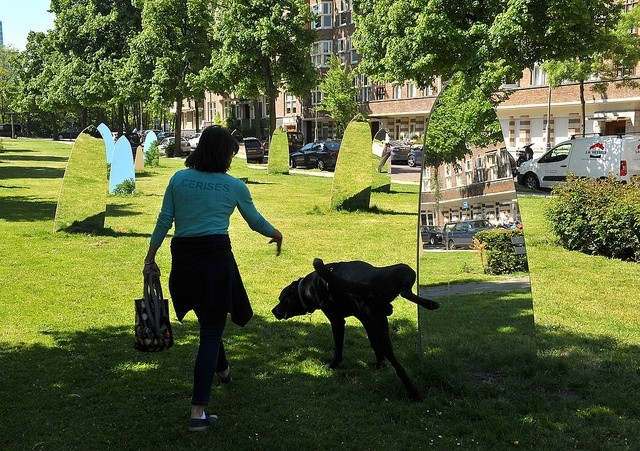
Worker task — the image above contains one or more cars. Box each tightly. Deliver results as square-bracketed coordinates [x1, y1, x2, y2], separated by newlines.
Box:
[158, 135, 191, 156]
[389, 138, 408, 163]
[119, 132, 140, 146]
[189, 132, 202, 148]
[290, 140, 340, 169]
[112, 131, 119, 141]
[58, 128, 79, 138]
[407, 143, 423, 166]
[420, 225, 443, 245]
[157, 131, 176, 144]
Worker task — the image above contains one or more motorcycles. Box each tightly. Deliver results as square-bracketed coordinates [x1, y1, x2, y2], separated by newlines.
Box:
[516, 141, 535, 164]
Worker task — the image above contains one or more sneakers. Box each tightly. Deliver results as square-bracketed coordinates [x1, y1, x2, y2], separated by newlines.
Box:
[216, 363, 233, 385]
[187, 410, 219, 432]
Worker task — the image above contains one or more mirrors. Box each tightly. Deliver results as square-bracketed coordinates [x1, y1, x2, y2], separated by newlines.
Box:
[225, 129, 248, 184]
[417, 72, 539, 402]
[331, 113, 372, 213]
[50, 125, 108, 236]
[97, 123, 114, 191]
[109, 135, 136, 197]
[268, 126, 289, 175]
[143, 131, 159, 167]
[371, 128, 392, 194]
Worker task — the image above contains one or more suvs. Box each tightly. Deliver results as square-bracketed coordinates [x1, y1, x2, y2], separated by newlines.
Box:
[244, 136, 265, 163]
[140, 129, 162, 141]
[285, 130, 306, 151]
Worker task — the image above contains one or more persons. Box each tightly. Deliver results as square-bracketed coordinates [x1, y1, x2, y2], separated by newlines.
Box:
[142, 123, 283, 432]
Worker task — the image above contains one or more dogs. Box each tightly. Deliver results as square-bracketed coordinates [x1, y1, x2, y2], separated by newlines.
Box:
[271, 257, 441, 404]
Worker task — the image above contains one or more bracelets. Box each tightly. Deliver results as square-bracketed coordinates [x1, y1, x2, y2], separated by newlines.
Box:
[144, 259, 155, 265]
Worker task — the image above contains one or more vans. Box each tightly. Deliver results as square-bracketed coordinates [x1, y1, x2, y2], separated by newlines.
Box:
[1, 124, 22, 138]
[443, 219, 495, 249]
[518, 131, 639, 191]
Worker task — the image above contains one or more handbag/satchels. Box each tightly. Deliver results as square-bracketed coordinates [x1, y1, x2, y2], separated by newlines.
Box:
[133, 271, 174, 353]
